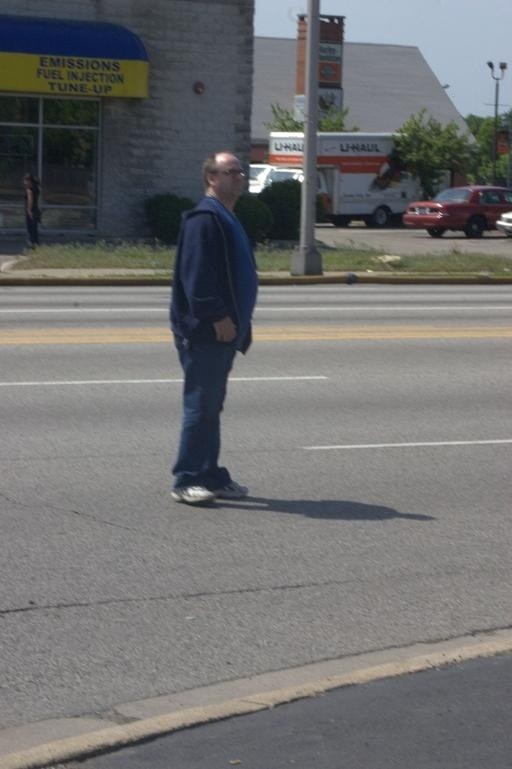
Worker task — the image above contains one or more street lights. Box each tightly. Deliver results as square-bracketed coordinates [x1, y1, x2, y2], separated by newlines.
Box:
[487, 59, 507, 185]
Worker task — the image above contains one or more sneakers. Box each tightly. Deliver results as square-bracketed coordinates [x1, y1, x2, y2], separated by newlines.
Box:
[213, 480, 249, 498]
[171, 486, 214, 504]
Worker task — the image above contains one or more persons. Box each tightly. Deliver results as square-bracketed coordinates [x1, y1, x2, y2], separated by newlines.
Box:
[21, 173, 42, 249]
[165, 150, 261, 510]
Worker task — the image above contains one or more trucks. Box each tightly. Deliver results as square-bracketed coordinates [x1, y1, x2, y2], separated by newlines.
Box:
[265, 128, 454, 229]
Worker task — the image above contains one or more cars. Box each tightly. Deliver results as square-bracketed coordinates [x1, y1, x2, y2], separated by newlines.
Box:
[403, 185, 510, 237]
[496, 210, 512, 236]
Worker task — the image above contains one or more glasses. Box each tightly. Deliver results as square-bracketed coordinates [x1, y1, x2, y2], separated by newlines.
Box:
[222, 169, 247, 177]
